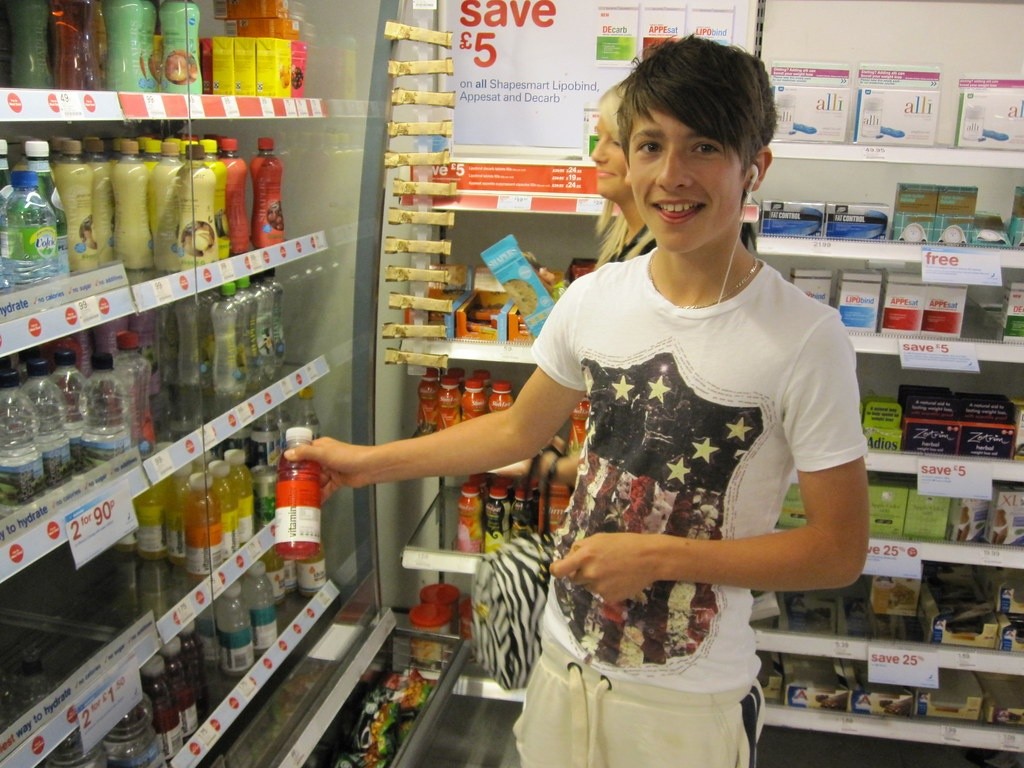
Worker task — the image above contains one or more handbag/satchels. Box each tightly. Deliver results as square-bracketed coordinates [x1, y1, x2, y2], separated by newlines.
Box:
[473, 532, 557, 691]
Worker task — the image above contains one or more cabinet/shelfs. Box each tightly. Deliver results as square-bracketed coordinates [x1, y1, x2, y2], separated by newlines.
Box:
[399, 128, 1023, 756]
[0, 85, 401, 768]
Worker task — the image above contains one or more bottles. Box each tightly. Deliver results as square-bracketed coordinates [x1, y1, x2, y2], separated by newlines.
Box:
[4, 0, 208, 95]
[0, 265, 305, 523]
[0, 136, 286, 286]
[0, 399, 331, 767]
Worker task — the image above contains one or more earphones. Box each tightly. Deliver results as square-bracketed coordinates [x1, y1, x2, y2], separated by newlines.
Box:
[747, 164, 759, 204]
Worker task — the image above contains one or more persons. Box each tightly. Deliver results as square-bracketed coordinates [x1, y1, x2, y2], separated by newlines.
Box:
[446, 74, 665, 497]
[276, 34, 869, 768]
